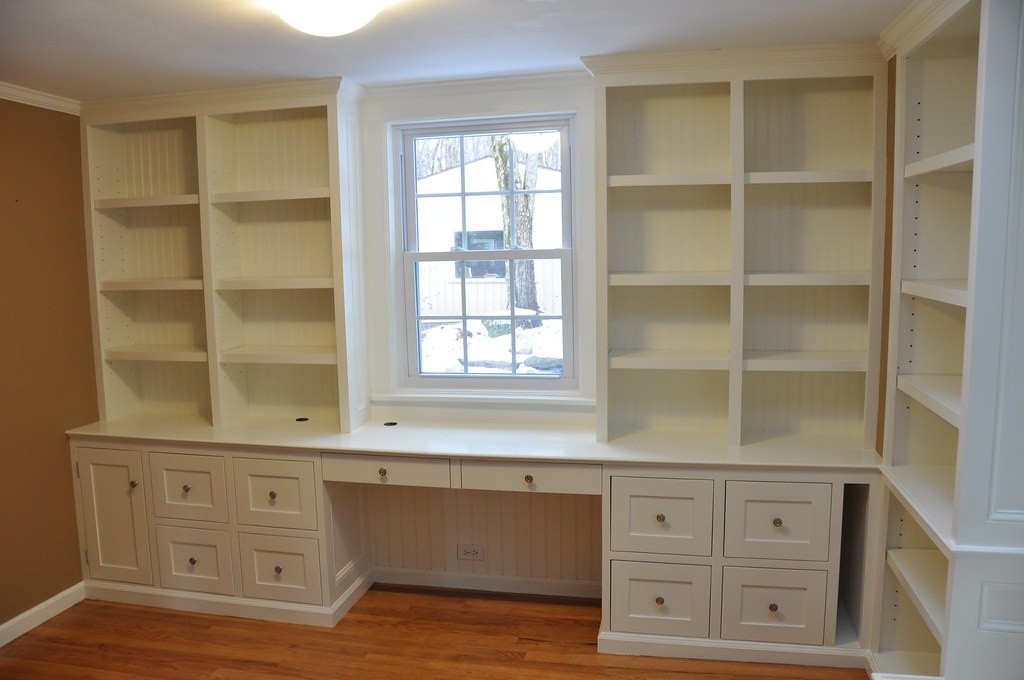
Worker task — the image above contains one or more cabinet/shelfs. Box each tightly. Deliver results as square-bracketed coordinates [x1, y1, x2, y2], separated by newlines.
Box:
[68, 436, 374, 629]
[593, 62, 888, 452]
[319, 423, 601, 496]
[602, 475, 845, 647]
[868, 0, 1023, 680]
[79, 92, 372, 434]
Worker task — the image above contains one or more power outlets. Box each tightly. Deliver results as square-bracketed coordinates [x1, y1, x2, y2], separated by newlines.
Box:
[458, 544, 485, 561]
